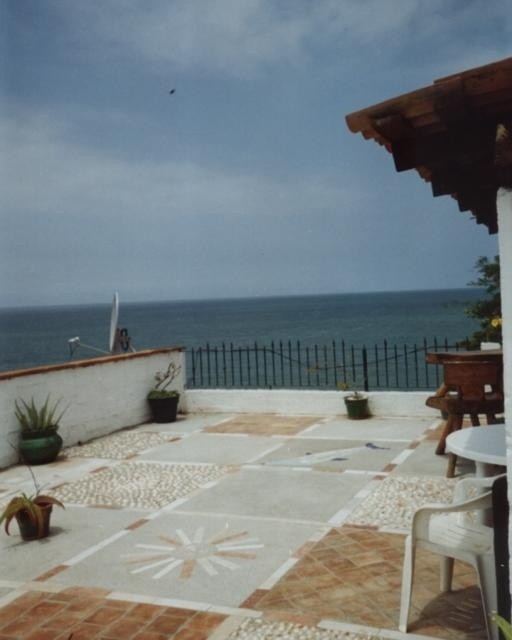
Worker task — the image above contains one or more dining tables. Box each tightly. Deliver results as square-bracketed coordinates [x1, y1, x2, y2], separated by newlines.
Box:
[0, 482, 66, 542]
[147, 360, 182, 423]
[4, 393, 72, 464]
[337, 375, 370, 420]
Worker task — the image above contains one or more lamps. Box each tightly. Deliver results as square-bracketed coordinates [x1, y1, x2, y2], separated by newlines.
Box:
[399, 473, 507, 640]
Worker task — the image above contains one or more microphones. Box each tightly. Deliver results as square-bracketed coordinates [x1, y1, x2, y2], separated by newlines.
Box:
[425, 394, 504, 478]
